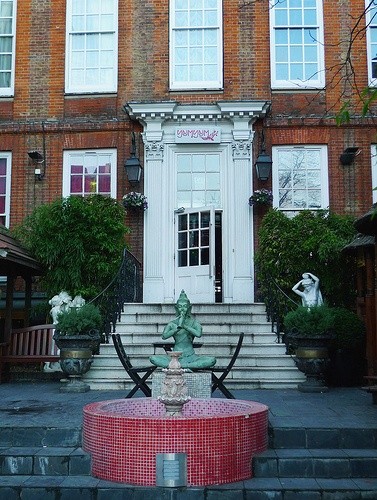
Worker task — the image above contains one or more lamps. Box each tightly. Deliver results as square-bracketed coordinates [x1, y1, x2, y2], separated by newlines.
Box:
[28, 150, 42, 162]
[124, 122, 144, 185]
[255, 119, 273, 183]
[340, 145, 361, 165]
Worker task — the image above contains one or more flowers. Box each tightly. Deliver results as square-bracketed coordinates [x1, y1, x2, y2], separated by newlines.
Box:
[248, 189, 274, 207]
[120, 193, 148, 210]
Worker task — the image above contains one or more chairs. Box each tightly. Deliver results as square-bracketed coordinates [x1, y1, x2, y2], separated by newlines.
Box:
[209, 332, 245, 399]
[111, 334, 157, 399]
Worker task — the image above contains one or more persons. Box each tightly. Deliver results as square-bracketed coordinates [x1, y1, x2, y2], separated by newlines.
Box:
[149, 289, 216, 369]
[43, 291, 86, 372]
[291, 272, 323, 311]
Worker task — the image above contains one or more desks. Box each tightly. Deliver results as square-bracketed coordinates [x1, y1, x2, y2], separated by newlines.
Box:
[153, 341, 205, 355]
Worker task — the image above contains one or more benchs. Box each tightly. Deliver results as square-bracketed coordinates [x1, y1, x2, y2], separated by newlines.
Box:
[1, 324, 60, 383]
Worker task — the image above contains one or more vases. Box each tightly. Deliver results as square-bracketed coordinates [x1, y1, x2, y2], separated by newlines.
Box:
[130, 203, 142, 212]
[256, 200, 269, 208]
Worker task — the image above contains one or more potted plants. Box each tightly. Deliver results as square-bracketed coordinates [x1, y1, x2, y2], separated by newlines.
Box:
[283, 305, 365, 392]
[55, 303, 104, 393]
